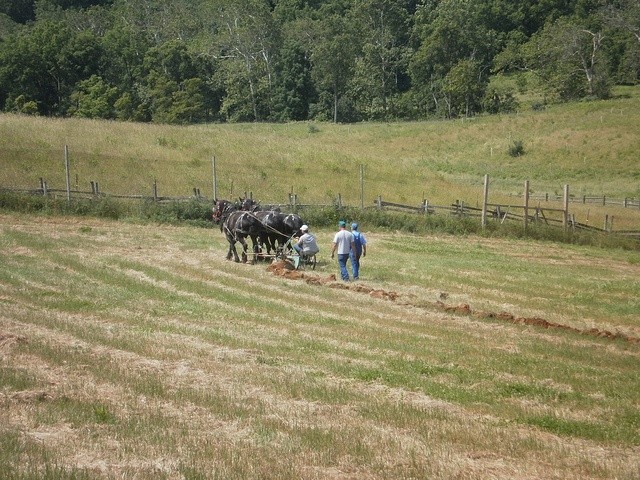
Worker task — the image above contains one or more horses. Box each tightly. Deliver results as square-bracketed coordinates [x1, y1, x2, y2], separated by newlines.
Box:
[212, 197, 261, 264]
[238, 195, 303, 254]
[235, 207, 284, 262]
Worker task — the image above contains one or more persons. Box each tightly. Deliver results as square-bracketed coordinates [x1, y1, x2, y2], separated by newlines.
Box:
[292, 224, 320, 260]
[330, 221, 357, 282]
[348, 222, 366, 279]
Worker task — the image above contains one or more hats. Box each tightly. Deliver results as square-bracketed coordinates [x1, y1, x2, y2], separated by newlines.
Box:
[351, 223, 359, 228]
[300, 225, 308, 230]
[338, 221, 345, 227]
